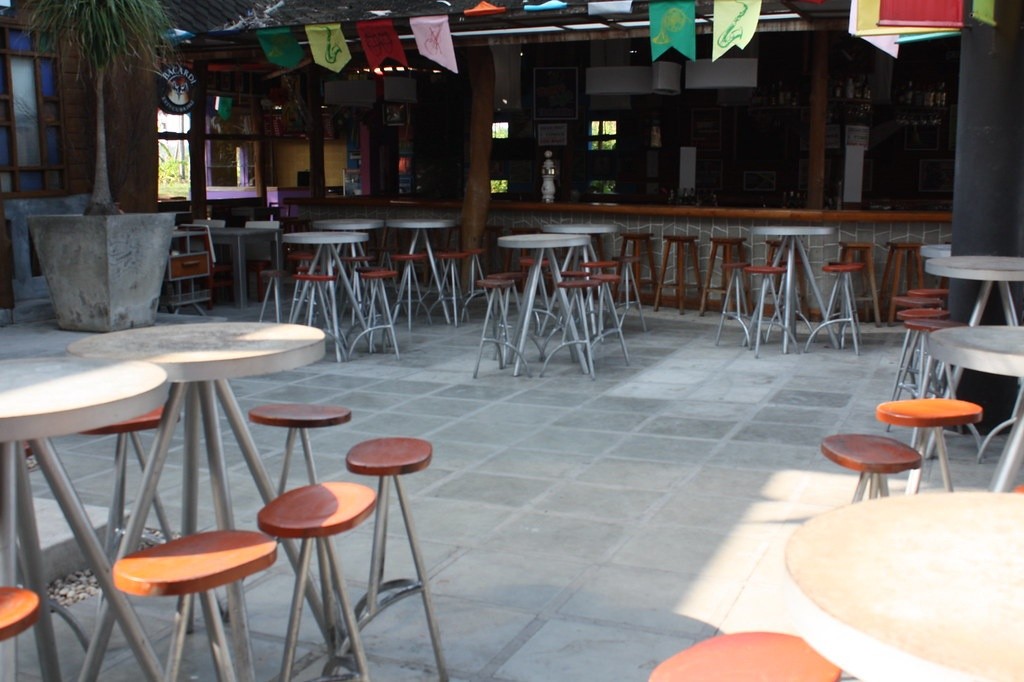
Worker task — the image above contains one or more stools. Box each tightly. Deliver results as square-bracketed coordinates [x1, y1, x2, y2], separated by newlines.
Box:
[0, 206, 985, 682]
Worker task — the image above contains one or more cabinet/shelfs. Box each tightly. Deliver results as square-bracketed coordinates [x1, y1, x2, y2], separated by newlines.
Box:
[161, 222, 216, 318]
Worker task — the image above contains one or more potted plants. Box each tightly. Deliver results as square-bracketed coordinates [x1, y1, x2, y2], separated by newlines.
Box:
[13, 1, 181, 331]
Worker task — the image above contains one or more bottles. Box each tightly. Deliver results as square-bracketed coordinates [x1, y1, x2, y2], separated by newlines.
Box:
[782, 191, 807, 208]
[825, 196, 836, 210]
[667, 188, 718, 206]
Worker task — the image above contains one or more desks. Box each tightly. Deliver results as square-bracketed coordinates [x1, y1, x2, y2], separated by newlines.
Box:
[919, 245, 952, 259]
[310, 218, 386, 327]
[497, 233, 591, 377]
[203, 186, 311, 222]
[539, 223, 618, 339]
[0, 358, 175, 682]
[742, 226, 840, 355]
[927, 325, 1024, 493]
[387, 220, 463, 326]
[67, 321, 337, 682]
[204, 228, 284, 310]
[778, 486, 1024, 682]
[281, 233, 377, 364]
[924, 256, 1023, 461]
